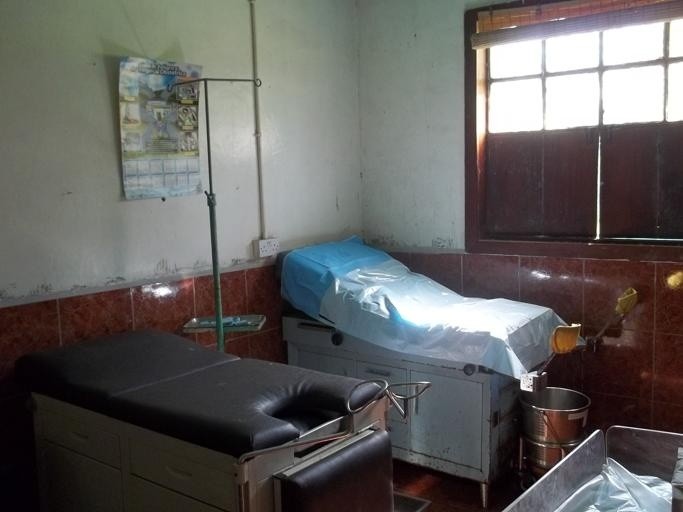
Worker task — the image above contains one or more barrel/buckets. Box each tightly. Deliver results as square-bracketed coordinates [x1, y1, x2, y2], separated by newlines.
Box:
[519, 386, 591, 474]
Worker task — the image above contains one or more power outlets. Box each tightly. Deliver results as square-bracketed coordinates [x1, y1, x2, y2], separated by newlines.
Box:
[253, 237, 288, 262]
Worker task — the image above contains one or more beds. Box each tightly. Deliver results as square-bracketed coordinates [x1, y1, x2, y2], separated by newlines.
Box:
[13, 319, 433, 512]
[274, 231, 640, 510]
